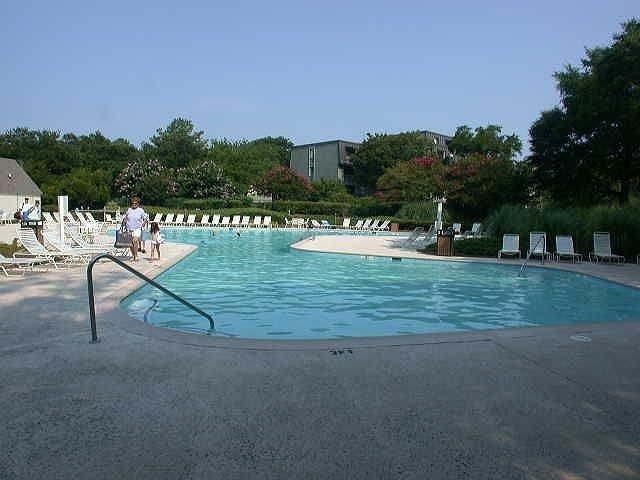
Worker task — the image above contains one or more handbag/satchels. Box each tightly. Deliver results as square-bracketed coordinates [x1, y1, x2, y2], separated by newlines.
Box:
[114, 229, 135, 249]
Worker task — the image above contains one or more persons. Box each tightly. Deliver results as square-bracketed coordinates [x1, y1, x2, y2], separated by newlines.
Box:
[21, 197, 32, 218]
[122, 197, 149, 263]
[149, 221, 164, 262]
[137, 214, 149, 254]
[27, 200, 40, 221]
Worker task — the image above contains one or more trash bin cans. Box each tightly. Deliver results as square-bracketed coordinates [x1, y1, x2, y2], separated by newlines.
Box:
[21, 219, 44, 246]
[437, 229, 455, 256]
[391, 223, 399, 232]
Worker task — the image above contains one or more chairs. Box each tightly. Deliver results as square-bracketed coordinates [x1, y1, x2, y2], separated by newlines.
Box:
[148, 213, 273, 229]
[0, 209, 131, 277]
[383, 225, 442, 251]
[283, 216, 336, 229]
[342, 218, 351, 228]
[497, 231, 626, 267]
[446, 222, 480, 240]
[352, 218, 391, 232]
